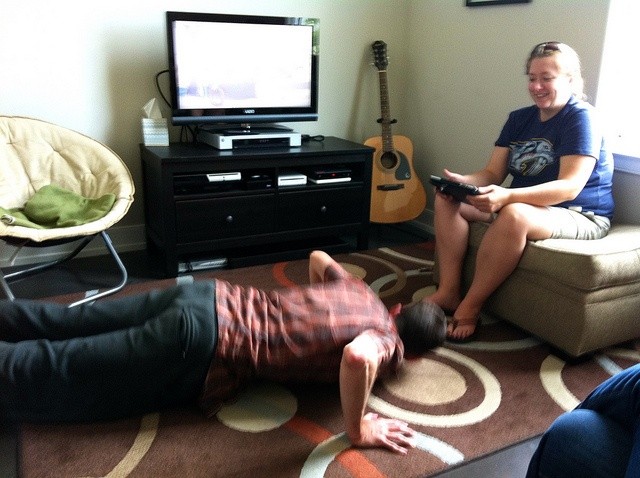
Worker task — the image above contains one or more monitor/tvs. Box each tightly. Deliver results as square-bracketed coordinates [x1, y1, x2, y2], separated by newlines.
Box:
[165, 11, 320, 132]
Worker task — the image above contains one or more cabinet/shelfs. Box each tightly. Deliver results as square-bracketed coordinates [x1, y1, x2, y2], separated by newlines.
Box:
[139, 136, 376, 276]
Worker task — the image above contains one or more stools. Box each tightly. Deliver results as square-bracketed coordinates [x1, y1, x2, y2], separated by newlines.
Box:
[433, 222, 640, 358]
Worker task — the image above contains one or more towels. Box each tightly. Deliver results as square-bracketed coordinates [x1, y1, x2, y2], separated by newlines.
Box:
[1, 185, 116, 229]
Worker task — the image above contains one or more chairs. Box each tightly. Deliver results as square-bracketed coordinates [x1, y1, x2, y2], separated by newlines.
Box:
[1, 117, 135, 308]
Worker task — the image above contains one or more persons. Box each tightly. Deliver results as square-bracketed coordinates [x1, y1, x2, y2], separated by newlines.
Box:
[1, 250, 448, 454]
[419, 41, 615, 344]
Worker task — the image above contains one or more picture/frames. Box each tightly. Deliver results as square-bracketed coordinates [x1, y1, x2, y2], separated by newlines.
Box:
[466, 0, 531, 6]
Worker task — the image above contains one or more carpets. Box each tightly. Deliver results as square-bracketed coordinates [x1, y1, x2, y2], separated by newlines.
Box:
[18, 240, 639, 477]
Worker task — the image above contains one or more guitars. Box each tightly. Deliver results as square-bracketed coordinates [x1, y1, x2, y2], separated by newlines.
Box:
[363, 39, 427, 225]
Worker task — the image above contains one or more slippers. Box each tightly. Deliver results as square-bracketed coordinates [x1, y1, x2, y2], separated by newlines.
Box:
[446, 315, 482, 344]
